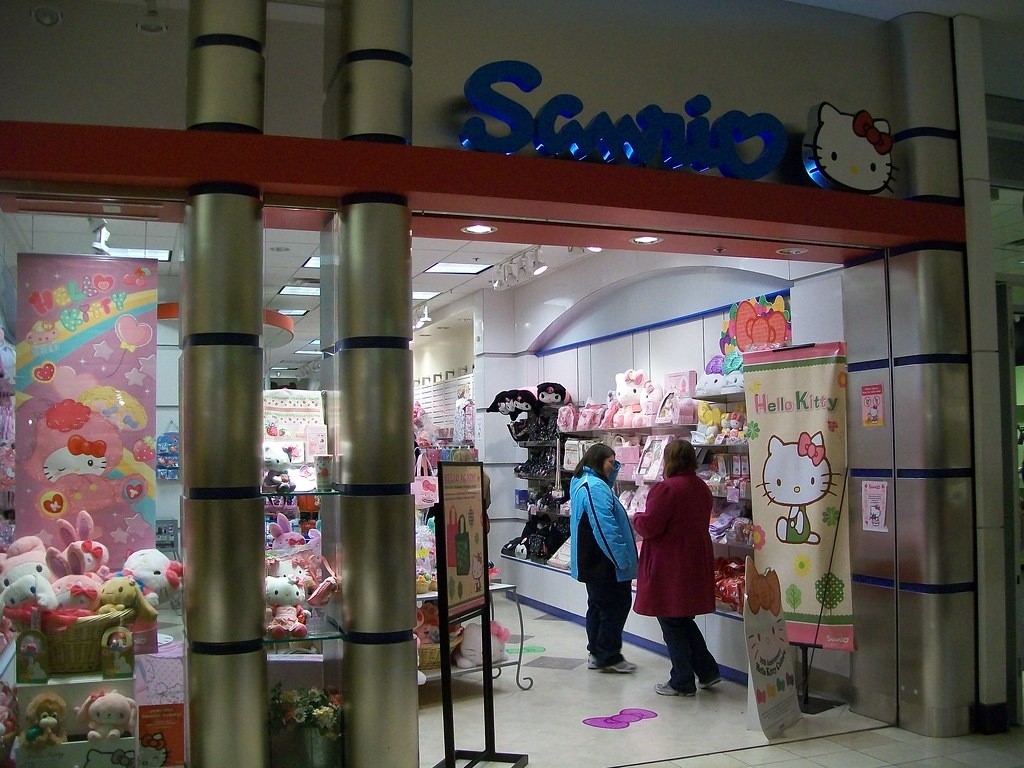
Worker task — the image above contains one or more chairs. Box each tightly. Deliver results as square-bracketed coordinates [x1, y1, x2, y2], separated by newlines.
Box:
[155, 520, 181, 562]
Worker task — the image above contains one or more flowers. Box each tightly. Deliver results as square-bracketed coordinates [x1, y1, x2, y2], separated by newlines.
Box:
[266, 682, 345, 742]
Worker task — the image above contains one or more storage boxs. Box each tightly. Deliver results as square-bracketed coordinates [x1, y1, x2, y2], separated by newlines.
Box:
[547, 536, 571, 569]
[613, 432, 681, 481]
[303, 425, 328, 463]
[564, 437, 602, 471]
[514, 489, 528, 509]
[643, 370, 696, 427]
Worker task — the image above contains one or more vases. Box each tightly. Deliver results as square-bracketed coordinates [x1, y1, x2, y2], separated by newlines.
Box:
[268, 726, 344, 768]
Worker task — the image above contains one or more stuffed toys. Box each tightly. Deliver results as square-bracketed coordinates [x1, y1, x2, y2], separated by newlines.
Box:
[19, 689, 138, 751]
[575, 369, 662, 430]
[705, 411, 748, 443]
[486, 382, 573, 564]
[263, 512, 324, 638]
[262, 446, 299, 493]
[695, 351, 745, 395]
[0, 509, 183, 621]
[412, 601, 510, 686]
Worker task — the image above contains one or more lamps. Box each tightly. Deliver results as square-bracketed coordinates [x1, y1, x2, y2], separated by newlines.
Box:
[492, 264, 502, 289]
[419, 304, 432, 322]
[532, 248, 547, 276]
[505, 259, 518, 287]
[90, 216, 112, 256]
[517, 252, 528, 282]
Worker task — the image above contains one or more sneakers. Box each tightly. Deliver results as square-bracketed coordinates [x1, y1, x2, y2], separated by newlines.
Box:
[587, 653, 599, 669]
[599, 660, 637, 673]
[697, 677, 721, 688]
[654, 682, 697, 697]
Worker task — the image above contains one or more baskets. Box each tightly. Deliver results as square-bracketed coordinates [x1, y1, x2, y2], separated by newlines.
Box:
[4, 602, 136, 673]
[419, 634, 465, 670]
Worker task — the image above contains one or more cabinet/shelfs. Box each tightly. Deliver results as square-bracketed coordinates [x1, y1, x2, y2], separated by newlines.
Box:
[518, 440, 570, 516]
[14, 671, 142, 767]
[263, 487, 345, 643]
[692, 388, 753, 547]
[553, 424, 697, 521]
[500, 549, 749, 686]
[416, 581, 534, 691]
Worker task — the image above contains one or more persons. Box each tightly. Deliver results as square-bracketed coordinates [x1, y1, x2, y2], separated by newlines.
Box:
[569, 442, 638, 673]
[630, 439, 722, 696]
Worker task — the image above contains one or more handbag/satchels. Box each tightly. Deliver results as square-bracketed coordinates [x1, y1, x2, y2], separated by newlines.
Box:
[447, 505, 459, 568]
[456, 514, 470, 575]
[412, 453, 439, 509]
